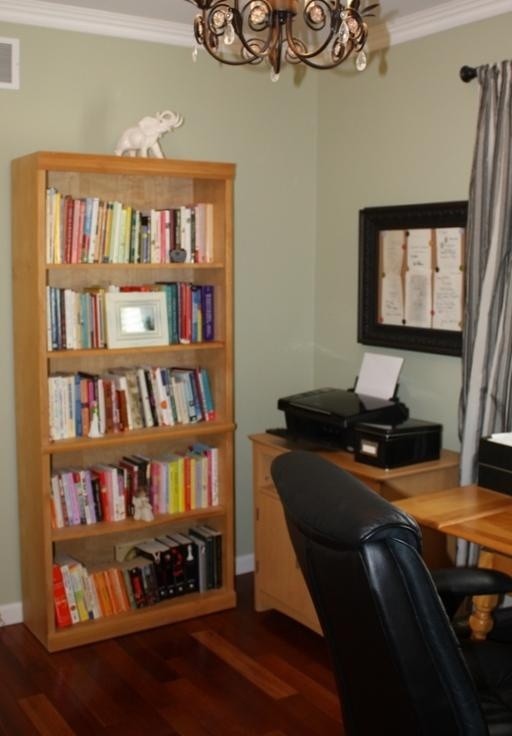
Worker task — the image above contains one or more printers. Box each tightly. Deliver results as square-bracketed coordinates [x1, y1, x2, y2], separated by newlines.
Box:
[276, 376, 408, 451]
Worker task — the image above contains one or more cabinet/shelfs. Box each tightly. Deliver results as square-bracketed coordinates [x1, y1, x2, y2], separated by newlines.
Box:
[248, 430, 469, 640]
[11, 149, 236, 655]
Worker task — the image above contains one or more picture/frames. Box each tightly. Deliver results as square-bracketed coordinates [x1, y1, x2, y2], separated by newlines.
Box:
[104, 291, 170, 351]
[356, 200, 468, 357]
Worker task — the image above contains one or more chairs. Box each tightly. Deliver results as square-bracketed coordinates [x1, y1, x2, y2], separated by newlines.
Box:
[270, 448, 511, 736]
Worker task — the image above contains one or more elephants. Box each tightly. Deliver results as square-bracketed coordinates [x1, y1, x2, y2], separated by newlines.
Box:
[114, 110, 184, 159]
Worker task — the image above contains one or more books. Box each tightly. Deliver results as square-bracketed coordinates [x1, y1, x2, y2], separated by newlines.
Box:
[51, 526, 223, 632]
[47, 366, 215, 440]
[45, 187, 215, 264]
[45, 281, 214, 352]
[50, 443, 220, 530]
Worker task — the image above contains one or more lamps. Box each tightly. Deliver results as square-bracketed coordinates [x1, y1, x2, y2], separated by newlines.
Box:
[185, 0, 379, 76]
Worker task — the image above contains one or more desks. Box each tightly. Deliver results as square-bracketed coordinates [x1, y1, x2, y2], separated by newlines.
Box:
[393, 484, 512, 643]
